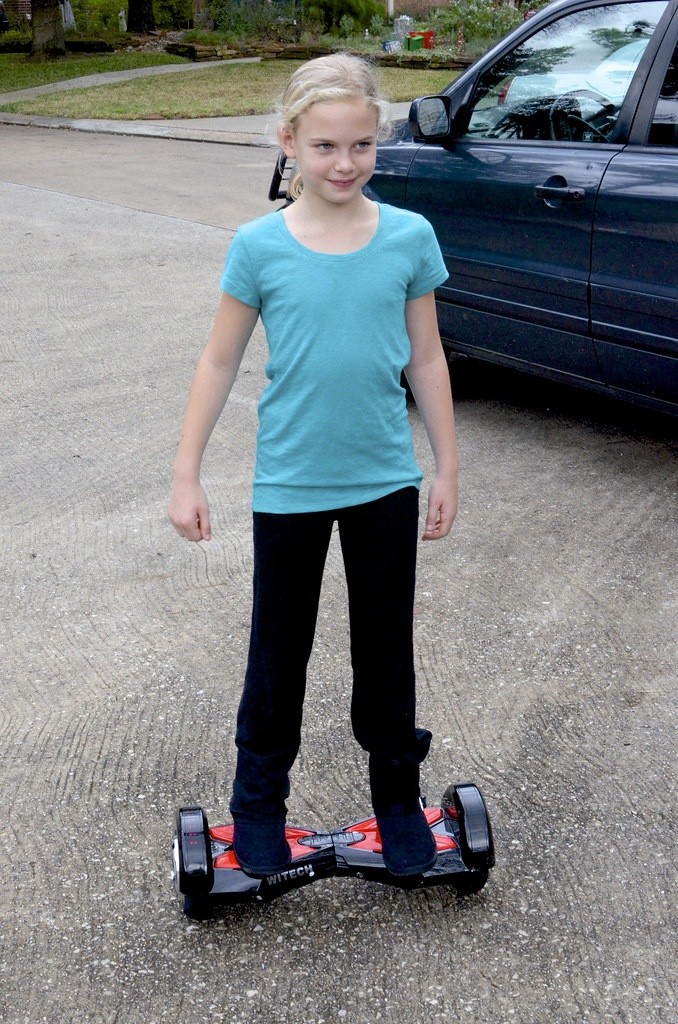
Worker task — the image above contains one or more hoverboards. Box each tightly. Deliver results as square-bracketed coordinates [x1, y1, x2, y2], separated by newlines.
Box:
[171, 779, 496, 928]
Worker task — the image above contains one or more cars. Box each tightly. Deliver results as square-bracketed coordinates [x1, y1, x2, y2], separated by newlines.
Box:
[268, 0, 678, 445]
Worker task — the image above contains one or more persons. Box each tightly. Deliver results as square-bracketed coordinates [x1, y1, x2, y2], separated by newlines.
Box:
[160, 55, 460, 883]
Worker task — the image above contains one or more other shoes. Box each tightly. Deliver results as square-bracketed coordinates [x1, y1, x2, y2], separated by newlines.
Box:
[375, 811, 438, 878]
[232, 822, 292, 878]
